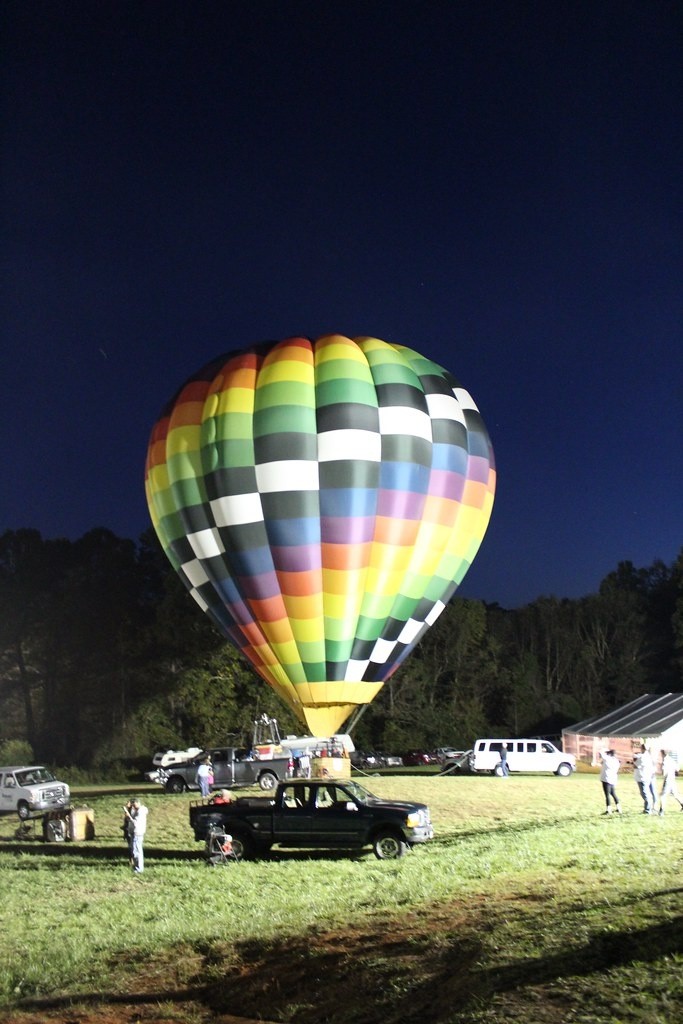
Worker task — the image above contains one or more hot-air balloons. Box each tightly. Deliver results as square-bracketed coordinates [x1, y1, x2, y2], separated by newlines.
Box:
[142, 331, 499, 781]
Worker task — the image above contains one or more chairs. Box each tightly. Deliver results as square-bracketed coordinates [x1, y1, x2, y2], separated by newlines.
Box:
[283, 791, 335, 808]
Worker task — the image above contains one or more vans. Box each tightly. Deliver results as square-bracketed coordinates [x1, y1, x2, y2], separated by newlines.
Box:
[0, 765, 71, 820]
[471, 738, 578, 777]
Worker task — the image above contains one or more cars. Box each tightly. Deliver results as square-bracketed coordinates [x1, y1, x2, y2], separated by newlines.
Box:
[441, 751, 474, 775]
[347, 745, 467, 770]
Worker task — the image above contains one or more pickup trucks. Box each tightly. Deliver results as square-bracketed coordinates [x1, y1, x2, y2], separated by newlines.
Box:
[144, 747, 295, 793]
[188, 779, 435, 861]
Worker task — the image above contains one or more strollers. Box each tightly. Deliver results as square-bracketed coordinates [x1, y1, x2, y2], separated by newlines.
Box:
[206, 822, 240, 869]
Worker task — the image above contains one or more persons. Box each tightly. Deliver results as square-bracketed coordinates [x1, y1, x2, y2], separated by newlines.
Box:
[500, 743, 509, 777]
[297, 747, 342, 778]
[195, 754, 215, 797]
[632, 744, 683, 817]
[598, 750, 624, 815]
[120, 798, 148, 872]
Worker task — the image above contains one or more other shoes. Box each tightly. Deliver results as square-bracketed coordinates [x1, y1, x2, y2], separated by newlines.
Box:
[643, 807, 665, 816]
[600, 808, 621, 815]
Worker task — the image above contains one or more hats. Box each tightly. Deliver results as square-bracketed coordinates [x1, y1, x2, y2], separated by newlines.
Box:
[130, 798, 140, 804]
[605, 749, 616, 755]
[641, 743, 649, 750]
